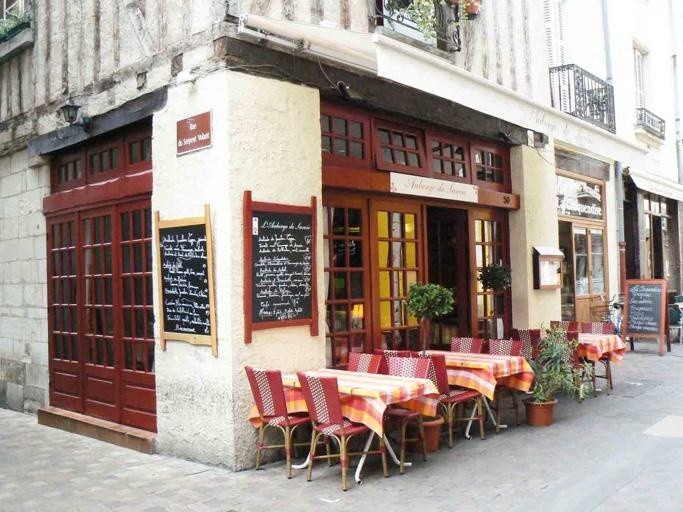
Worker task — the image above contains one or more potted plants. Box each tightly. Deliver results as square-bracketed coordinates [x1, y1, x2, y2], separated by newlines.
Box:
[666, 306, 682, 344]
[524, 331, 593, 428]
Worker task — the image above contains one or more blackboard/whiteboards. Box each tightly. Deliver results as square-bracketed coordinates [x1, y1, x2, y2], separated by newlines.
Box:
[154, 218, 216, 346]
[623, 279, 666, 338]
[242, 201, 318, 330]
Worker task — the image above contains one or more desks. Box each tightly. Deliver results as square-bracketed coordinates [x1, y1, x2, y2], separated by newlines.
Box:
[420, 349, 535, 439]
[275, 365, 439, 487]
[449, 321, 613, 434]
[541, 332, 628, 397]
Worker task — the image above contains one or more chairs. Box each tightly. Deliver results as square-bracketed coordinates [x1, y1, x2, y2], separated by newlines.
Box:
[348, 351, 383, 374]
[296, 372, 390, 492]
[380, 357, 431, 476]
[373, 348, 410, 375]
[243, 366, 332, 479]
[410, 352, 486, 449]
